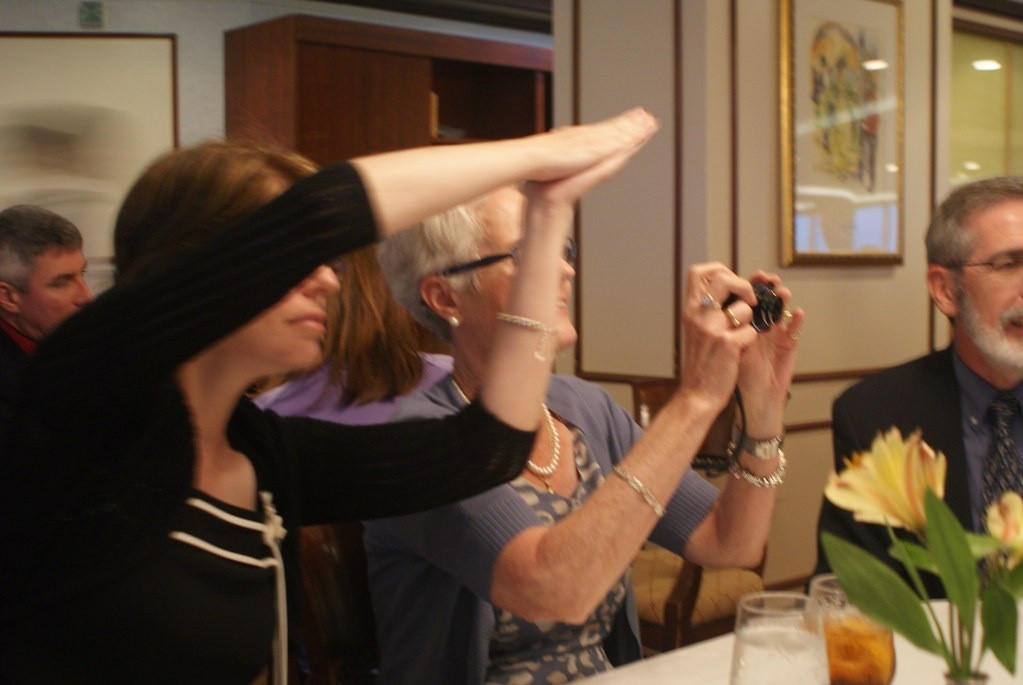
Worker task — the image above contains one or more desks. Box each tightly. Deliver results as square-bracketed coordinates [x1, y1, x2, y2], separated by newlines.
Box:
[560, 593, 1023, 685]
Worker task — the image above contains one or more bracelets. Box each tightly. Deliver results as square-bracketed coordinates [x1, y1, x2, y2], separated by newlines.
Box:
[724, 442, 785, 488]
[612, 465, 666, 517]
[735, 425, 784, 459]
[494, 313, 557, 334]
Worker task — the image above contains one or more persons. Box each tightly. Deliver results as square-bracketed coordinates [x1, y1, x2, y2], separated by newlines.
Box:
[365, 182, 803, 685]
[0, 106, 657, 685]
[0, 204, 95, 375]
[814, 179, 1023, 600]
[249, 247, 454, 424]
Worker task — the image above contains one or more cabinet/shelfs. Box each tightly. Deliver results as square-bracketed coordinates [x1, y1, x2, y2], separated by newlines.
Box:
[223, 12, 554, 168]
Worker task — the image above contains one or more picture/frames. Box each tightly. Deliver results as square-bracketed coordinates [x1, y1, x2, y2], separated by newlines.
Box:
[777, 0, 906, 271]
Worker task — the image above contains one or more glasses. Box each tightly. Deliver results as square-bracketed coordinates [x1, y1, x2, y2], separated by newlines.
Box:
[945, 251, 1023, 277]
[419, 237, 576, 309]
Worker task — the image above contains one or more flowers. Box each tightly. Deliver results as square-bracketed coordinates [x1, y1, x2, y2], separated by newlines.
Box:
[820, 431, 1023, 675]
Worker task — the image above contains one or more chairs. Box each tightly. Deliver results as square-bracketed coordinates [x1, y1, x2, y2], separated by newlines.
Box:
[606, 373, 768, 657]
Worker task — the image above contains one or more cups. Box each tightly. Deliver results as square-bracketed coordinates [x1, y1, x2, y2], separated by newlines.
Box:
[727, 592, 831, 685]
[809, 575, 896, 685]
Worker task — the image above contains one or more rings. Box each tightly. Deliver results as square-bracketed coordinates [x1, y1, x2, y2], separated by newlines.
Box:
[786, 324, 800, 342]
[701, 290, 721, 311]
[777, 310, 791, 322]
[722, 306, 739, 328]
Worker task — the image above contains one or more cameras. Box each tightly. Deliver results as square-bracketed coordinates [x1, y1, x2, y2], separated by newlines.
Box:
[721, 278, 783, 332]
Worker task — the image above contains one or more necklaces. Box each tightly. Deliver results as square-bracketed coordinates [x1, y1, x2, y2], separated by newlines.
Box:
[451, 382, 559, 479]
[538, 416, 556, 494]
